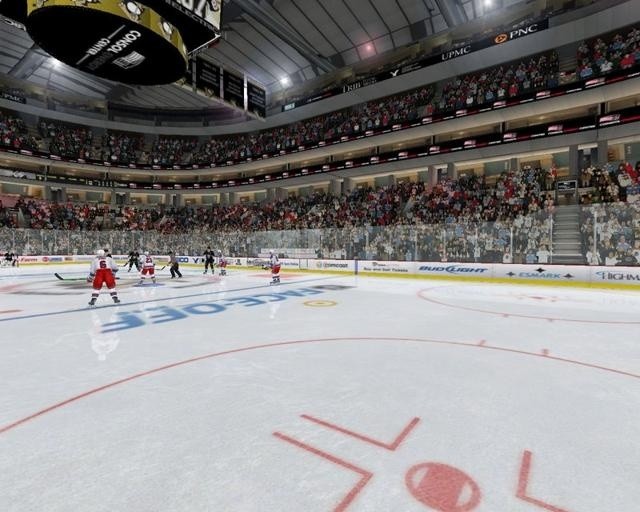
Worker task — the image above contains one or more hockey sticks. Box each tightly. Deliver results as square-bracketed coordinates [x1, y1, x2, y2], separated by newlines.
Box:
[141, 266, 165, 271]
[55, 272, 120, 280]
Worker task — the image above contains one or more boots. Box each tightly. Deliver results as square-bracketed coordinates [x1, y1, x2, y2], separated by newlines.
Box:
[88, 298, 97, 305]
[112, 297, 120, 303]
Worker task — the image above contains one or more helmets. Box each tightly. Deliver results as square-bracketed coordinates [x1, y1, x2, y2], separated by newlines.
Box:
[98, 250, 105, 257]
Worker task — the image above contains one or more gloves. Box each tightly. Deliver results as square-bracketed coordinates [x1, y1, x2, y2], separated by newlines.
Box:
[87, 273, 95, 282]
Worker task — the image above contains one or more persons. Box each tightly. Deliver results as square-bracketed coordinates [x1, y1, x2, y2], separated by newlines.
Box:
[0, 28, 640, 266]
[89, 249, 120, 305]
[127, 248, 156, 284]
[202, 246, 227, 275]
[166, 251, 182, 279]
[268, 249, 281, 285]
